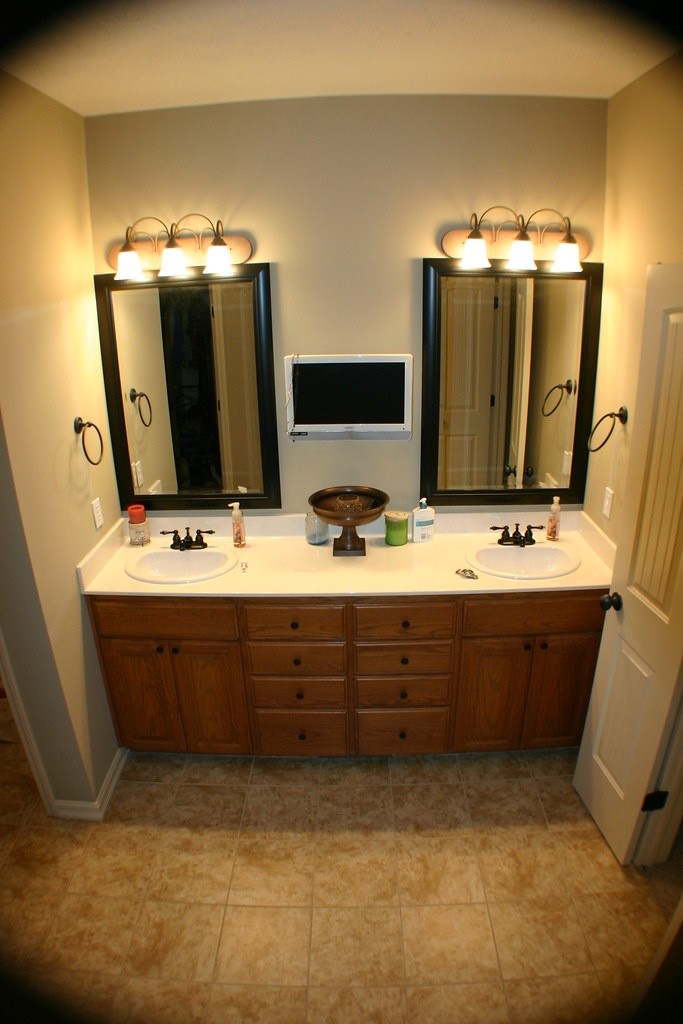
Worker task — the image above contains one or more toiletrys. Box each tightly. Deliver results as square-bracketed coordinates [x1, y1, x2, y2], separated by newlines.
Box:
[228, 502, 247, 548]
[411, 498, 435, 543]
[545, 496, 560, 541]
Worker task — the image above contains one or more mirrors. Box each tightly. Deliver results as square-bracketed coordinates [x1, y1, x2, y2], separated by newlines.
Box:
[418, 257, 604, 506]
[93, 262, 283, 510]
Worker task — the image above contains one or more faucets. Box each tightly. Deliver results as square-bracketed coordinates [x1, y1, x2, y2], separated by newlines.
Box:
[159, 526, 215, 552]
[489, 522, 545, 547]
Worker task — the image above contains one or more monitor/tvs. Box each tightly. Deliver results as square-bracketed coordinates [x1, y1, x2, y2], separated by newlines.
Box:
[283, 353, 412, 443]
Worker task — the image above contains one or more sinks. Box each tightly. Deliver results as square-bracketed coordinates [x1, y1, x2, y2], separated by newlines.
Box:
[464, 543, 582, 580]
[124, 546, 239, 584]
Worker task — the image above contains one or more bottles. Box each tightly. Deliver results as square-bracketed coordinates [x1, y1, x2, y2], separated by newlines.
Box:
[305, 512, 329, 545]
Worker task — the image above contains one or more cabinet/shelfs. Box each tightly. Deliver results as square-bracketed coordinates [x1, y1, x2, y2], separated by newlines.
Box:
[86, 589, 610, 758]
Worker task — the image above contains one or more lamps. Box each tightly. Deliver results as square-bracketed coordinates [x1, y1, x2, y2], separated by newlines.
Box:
[106, 219, 252, 280]
[442, 212, 593, 273]
